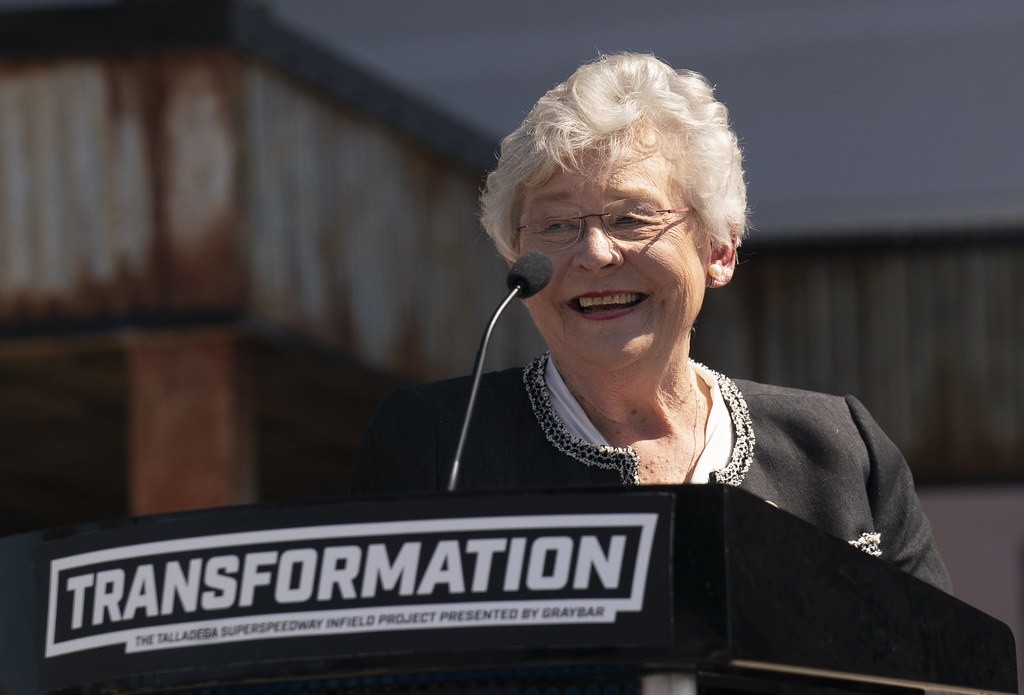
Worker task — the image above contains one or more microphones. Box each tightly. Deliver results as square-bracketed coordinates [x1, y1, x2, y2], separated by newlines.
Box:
[447, 250, 553, 491]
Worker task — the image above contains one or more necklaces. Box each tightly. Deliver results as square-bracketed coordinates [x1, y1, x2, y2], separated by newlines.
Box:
[575, 393, 698, 482]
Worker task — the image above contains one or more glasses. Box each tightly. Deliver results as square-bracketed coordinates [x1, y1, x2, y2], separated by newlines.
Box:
[516, 196, 692, 252]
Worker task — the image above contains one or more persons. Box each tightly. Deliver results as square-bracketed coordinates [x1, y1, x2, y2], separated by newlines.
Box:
[346, 52, 954, 599]
